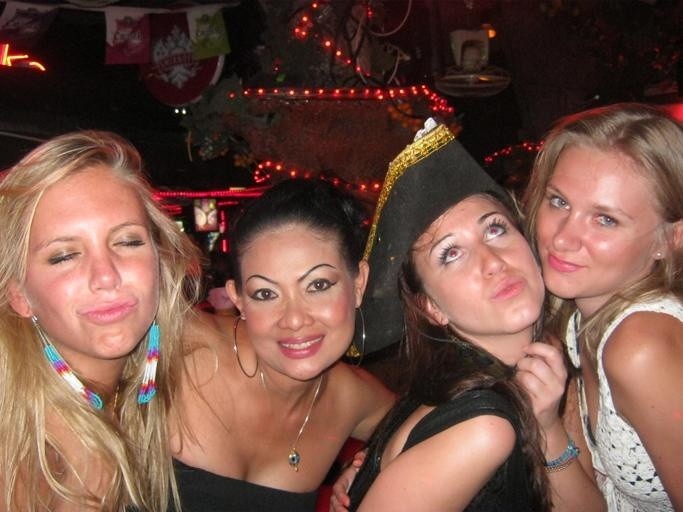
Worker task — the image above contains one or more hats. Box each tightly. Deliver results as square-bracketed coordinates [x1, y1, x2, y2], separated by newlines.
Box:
[346, 122, 522, 359]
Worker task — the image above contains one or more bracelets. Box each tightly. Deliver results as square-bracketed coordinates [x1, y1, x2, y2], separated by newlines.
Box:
[545, 440, 580, 474]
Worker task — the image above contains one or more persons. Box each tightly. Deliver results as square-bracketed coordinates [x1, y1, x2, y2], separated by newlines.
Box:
[343, 119, 606, 512]
[1, 128, 165, 511]
[165, 175, 400, 512]
[326, 106, 683, 509]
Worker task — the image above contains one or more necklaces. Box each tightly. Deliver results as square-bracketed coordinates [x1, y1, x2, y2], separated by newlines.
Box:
[258, 366, 323, 467]
